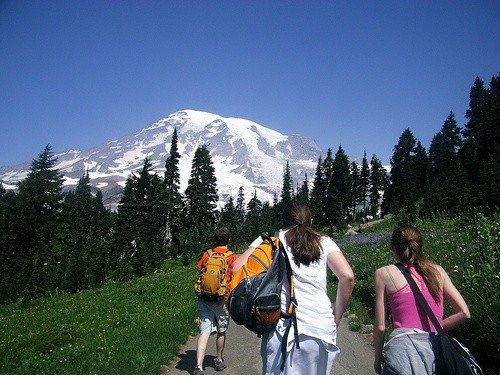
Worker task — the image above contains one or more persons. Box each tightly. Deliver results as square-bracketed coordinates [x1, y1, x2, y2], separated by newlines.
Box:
[232, 201, 354, 375]
[187, 227, 238, 375]
[373, 225, 470, 375]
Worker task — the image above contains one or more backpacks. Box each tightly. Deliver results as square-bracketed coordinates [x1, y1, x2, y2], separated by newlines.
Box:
[224, 230, 301, 373]
[200, 248, 233, 296]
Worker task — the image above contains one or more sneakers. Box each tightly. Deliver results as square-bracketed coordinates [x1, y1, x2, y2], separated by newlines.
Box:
[213, 358, 226, 371]
[190, 368, 204, 375]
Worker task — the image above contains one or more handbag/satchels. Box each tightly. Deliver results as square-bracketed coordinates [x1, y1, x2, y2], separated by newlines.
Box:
[439, 334, 483, 375]
